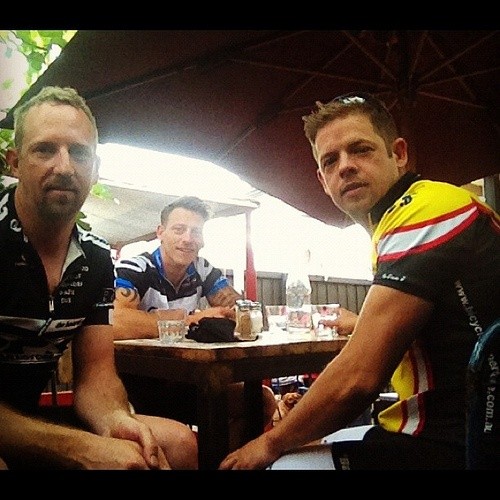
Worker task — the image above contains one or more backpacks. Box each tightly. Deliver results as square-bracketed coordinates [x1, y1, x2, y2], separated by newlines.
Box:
[463, 322, 500, 479]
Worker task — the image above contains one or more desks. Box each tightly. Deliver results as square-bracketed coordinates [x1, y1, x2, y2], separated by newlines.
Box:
[114, 335, 349, 471]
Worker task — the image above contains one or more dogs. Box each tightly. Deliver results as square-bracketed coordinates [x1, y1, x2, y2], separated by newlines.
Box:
[272, 392, 302, 426]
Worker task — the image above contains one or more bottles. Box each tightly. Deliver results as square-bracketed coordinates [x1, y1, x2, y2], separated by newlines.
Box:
[286, 252, 312, 339]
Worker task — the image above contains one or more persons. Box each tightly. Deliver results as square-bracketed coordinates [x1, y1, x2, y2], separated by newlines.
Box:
[112, 196, 242, 341]
[0, 86, 199, 472]
[215, 93, 498, 471]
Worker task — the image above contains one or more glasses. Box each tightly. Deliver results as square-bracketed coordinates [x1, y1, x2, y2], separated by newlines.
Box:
[331, 92, 390, 118]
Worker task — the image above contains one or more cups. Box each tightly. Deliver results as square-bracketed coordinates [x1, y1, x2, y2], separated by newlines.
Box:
[313, 303, 340, 340]
[156, 308, 185, 344]
[233, 299, 263, 341]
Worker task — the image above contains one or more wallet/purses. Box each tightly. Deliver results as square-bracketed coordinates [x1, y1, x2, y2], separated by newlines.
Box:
[185, 317, 236, 344]
